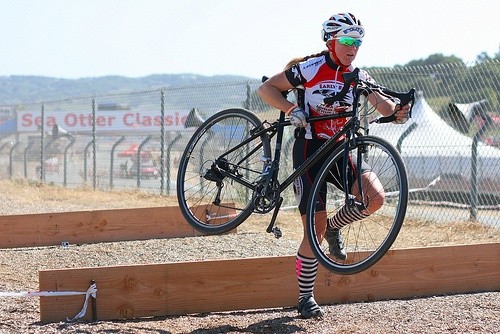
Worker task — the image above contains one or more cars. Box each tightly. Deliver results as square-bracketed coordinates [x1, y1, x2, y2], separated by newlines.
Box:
[129, 159, 157, 180]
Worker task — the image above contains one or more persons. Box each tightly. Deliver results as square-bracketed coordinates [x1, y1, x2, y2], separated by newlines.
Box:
[257, 12, 412, 319]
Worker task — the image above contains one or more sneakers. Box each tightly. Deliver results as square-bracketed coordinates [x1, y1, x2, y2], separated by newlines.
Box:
[297, 295, 324, 319]
[323, 218, 347, 262]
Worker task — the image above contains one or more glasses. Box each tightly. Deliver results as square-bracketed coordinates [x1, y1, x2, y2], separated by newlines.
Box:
[338, 37, 363, 46]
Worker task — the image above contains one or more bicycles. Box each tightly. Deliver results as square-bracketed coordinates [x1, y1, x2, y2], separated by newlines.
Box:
[176, 75, 415, 275]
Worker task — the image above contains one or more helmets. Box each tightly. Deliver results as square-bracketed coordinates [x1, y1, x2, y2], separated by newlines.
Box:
[321, 13, 365, 42]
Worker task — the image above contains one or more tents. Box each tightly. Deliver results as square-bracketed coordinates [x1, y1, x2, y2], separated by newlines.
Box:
[351, 87, 500, 205]
